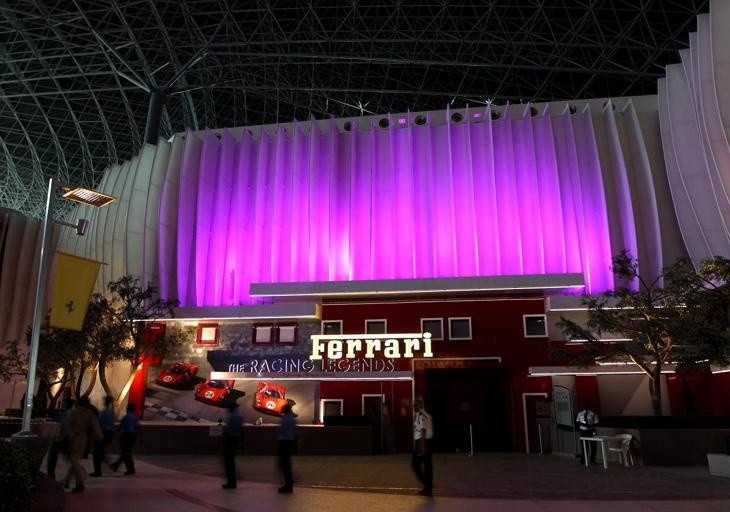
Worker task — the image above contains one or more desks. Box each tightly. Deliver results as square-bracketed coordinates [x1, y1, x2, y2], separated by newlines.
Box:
[580, 435, 629, 469]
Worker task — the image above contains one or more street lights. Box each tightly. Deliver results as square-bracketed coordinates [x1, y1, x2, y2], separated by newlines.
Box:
[22, 177, 117, 432]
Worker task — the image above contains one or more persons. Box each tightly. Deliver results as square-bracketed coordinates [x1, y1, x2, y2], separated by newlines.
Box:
[218, 401, 244, 490]
[275, 403, 296, 494]
[575, 401, 599, 464]
[410, 399, 435, 499]
[20, 393, 140, 495]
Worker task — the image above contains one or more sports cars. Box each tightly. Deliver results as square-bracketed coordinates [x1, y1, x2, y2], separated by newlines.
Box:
[253, 381, 290, 417]
[193, 375, 235, 405]
[154, 361, 199, 387]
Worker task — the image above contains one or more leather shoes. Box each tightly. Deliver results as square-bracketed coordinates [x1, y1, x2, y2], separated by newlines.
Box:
[72, 483, 85, 493]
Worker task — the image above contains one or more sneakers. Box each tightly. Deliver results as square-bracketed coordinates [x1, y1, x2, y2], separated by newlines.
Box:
[222, 484, 237, 489]
[89, 471, 102, 477]
[278, 486, 292, 493]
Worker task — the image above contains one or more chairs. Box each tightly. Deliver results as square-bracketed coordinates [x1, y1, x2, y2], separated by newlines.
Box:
[607, 434, 634, 467]
